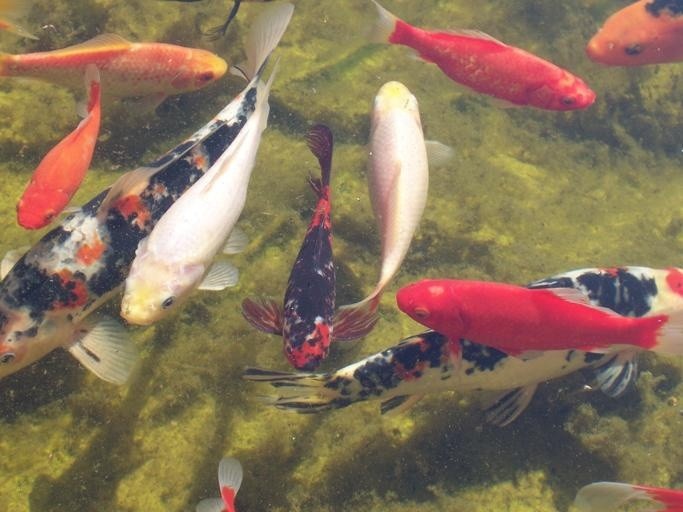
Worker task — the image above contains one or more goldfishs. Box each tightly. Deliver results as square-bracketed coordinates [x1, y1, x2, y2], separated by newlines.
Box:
[240, 268, 683, 428]
[0, 0, 431, 386]
